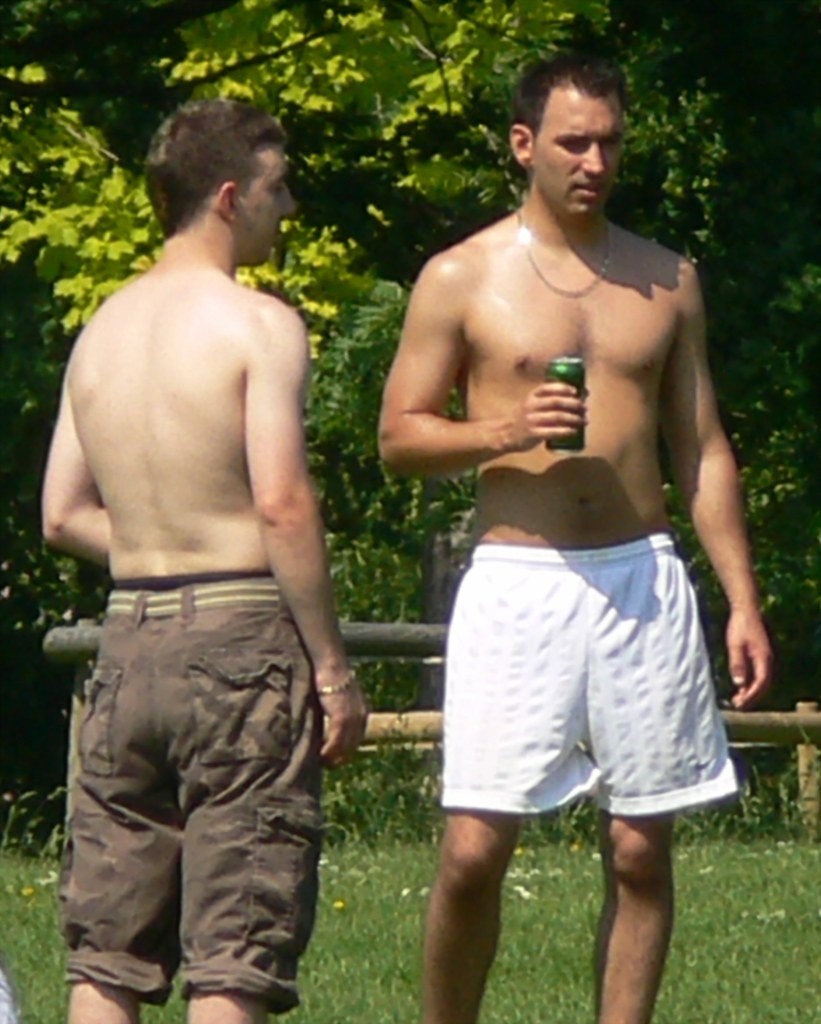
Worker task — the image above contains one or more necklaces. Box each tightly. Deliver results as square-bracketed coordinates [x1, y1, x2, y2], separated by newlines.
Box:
[517, 207, 612, 296]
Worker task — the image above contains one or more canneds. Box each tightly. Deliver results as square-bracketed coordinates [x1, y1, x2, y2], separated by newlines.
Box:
[546, 358, 586, 452]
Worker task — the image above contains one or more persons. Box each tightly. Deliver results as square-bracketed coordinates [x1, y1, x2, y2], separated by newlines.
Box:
[42, 98, 364, 1024]
[375, 49, 771, 1023]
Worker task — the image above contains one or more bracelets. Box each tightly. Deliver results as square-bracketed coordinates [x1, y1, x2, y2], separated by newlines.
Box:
[317, 669, 357, 694]
